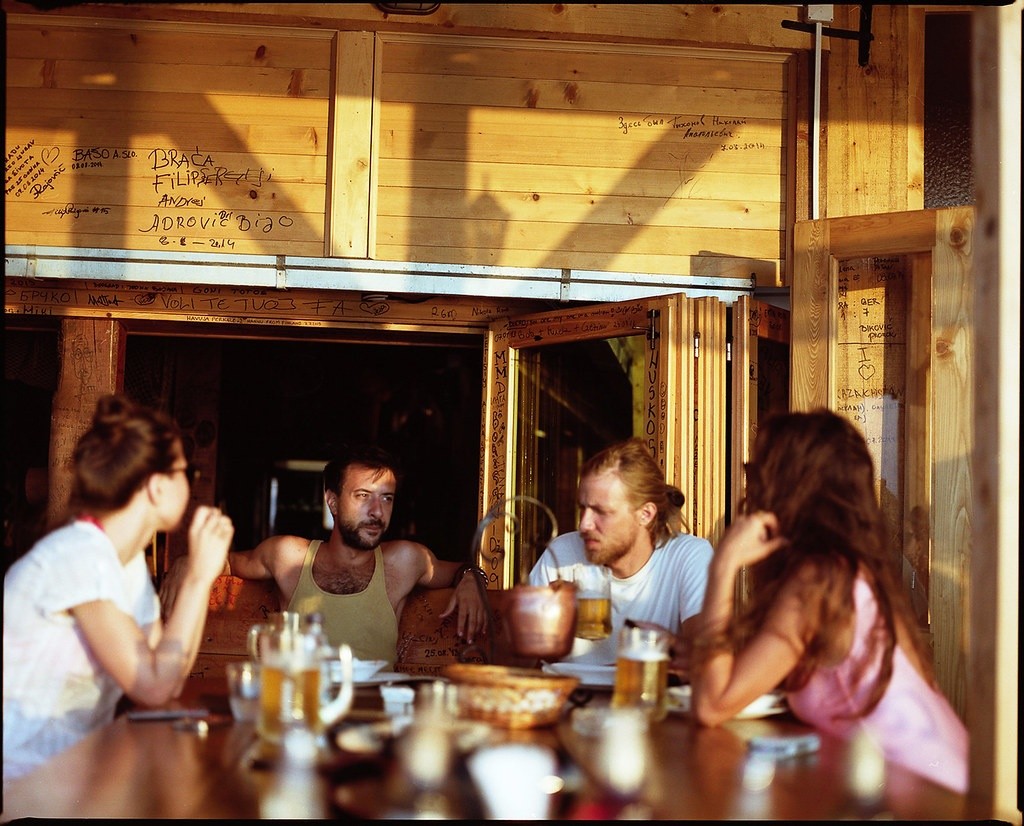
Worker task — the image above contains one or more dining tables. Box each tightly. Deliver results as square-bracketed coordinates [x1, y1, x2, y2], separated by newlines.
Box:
[2, 674, 1005, 821]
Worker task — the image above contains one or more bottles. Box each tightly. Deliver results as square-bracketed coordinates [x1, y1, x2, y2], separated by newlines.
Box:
[574, 712, 661, 821]
[308, 614, 328, 700]
[831, 736, 901, 819]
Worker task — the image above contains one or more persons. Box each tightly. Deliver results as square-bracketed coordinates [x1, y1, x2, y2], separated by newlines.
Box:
[158, 442, 490, 667]
[523, 440, 715, 678]
[693, 408, 970, 800]
[3, 393, 236, 785]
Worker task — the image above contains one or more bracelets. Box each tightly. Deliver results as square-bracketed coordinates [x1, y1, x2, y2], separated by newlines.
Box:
[461, 566, 490, 589]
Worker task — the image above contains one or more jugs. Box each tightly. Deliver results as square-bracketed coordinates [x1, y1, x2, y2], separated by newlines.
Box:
[245, 610, 354, 739]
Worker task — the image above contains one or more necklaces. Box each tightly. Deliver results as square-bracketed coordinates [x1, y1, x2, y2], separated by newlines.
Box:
[76, 514, 105, 532]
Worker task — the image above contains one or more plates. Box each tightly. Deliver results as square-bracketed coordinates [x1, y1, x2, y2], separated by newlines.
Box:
[338, 723, 491, 750]
[332, 671, 410, 688]
[542, 665, 614, 691]
[662, 704, 785, 720]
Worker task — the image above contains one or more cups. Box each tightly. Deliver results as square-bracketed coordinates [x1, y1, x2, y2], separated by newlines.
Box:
[574, 564, 610, 639]
[614, 629, 667, 717]
[225, 660, 260, 720]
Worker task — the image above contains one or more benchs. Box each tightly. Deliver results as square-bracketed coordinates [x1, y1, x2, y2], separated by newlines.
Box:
[183, 575, 541, 675]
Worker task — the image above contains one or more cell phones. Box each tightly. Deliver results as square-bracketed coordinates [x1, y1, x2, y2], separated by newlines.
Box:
[745, 732, 823, 759]
[127, 708, 210, 723]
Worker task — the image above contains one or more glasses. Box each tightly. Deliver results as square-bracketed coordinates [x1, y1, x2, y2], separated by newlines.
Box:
[160, 466, 201, 483]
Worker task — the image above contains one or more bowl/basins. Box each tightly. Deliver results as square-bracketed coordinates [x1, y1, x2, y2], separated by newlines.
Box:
[329, 660, 389, 682]
[551, 662, 616, 681]
[670, 687, 780, 710]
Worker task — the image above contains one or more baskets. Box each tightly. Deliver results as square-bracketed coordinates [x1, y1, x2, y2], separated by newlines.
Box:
[440, 664, 580, 727]
